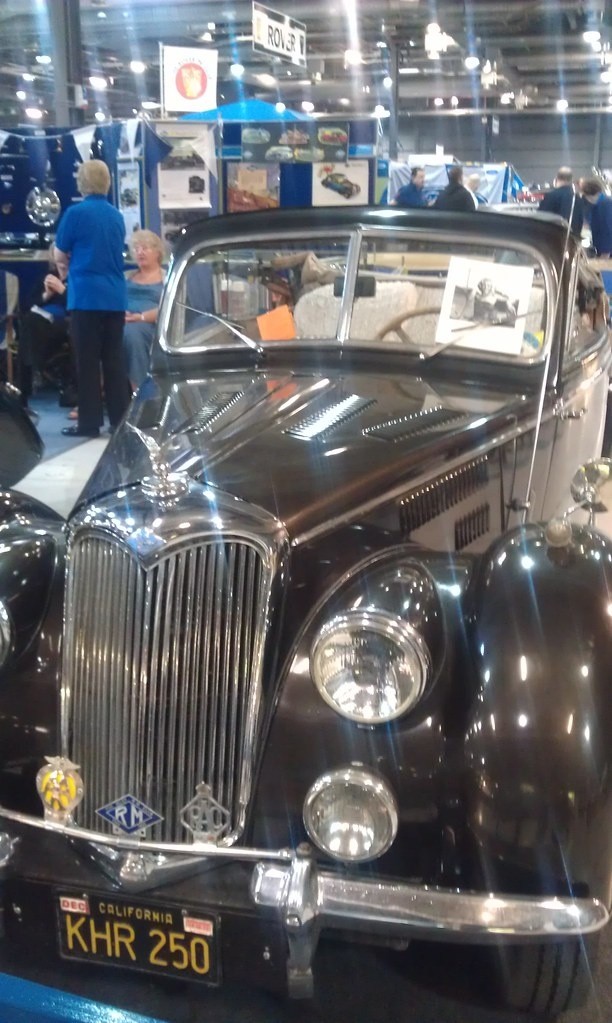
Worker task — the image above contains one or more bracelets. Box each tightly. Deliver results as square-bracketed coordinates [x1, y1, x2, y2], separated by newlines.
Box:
[140, 313, 144, 321]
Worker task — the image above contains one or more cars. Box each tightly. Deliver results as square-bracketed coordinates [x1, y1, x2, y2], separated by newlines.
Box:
[0, 201, 612, 1023]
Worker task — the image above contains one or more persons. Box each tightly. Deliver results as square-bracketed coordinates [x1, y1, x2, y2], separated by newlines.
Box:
[430, 165, 487, 212]
[473, 278, 515, 322]
[69, 229, 171, 419]
[389, 168, 428, 208]
[535, 167, 582, 234]
[52, 159, 131, 436]
[578, 177, 612, 317]
[16, 242, 67, 407]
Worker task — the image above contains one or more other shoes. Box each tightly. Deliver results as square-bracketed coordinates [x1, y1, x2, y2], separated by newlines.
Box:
[62, 424, 98, 436]
[68, 403, 78, 420]
[108, 426, 118, 434]
